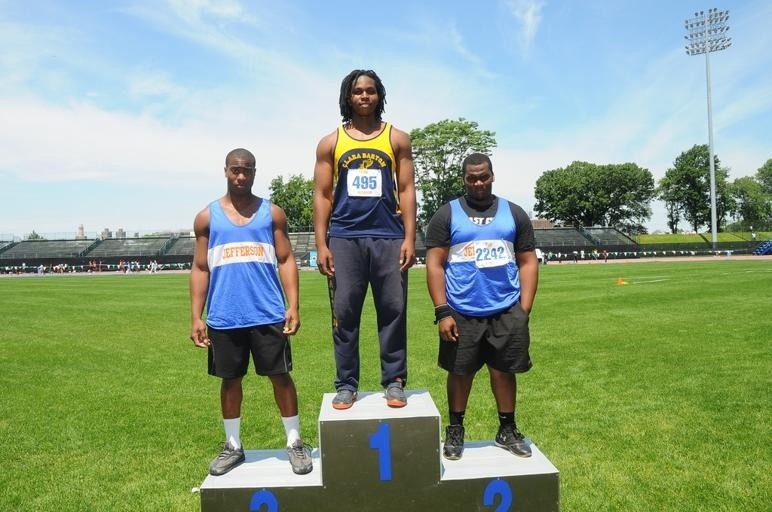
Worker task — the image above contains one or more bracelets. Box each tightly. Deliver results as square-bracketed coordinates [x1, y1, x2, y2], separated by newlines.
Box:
[434, 304, 453, 325]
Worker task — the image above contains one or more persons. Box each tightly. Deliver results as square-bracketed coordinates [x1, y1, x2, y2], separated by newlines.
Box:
[4, 257, 158, 274]
[751, 230, 757, 240]
[424, 154, 538, 460]
[542, 248, 608, 264]
[315, 70, 416, 409]
[191, 149, 312, 476]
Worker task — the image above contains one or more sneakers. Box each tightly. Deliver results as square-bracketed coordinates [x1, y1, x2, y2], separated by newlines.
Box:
[494, 425, 532, 458]
[286, 439, 313, 476]
[384, 381, 408, 407]
[331, 389, 358, 409]
[209, 440, 246, 477]
[443, 424, 466, 461]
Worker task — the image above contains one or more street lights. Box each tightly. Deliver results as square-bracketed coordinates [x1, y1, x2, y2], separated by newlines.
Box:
[685, 6, 733, 249]
